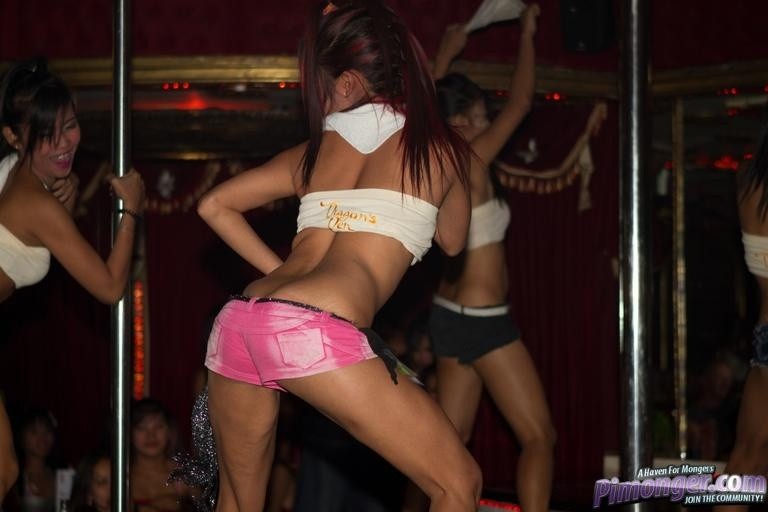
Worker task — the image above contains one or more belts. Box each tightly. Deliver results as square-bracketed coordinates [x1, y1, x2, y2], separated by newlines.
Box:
[431, 292, 511, 317]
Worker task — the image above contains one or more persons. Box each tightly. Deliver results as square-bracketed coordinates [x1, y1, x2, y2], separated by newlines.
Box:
[0, 0, 768, 511]
